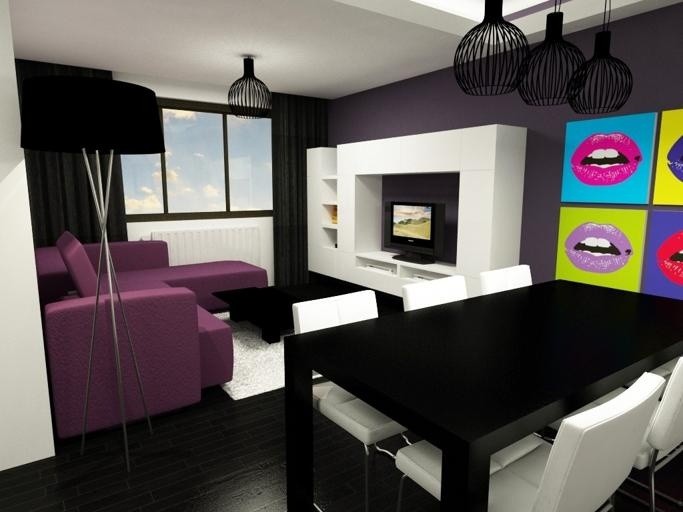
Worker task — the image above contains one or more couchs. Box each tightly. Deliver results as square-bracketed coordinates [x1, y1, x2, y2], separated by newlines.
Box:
[32, 231, 268, 443]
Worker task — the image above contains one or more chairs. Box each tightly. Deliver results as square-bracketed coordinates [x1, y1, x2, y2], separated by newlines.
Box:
[291, 289, 411, 511]
[403, 274, 470, 310]
[480, 264, 535, 294]
[549, 357, 682, 510]
[391, 369, 665, 510]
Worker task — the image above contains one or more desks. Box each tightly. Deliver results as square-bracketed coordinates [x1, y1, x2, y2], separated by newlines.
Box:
[281, 279, 680, 509]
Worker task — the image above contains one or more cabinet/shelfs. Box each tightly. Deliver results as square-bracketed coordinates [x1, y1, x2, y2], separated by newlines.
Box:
[306, 145, 339, 252]
[355, 254, 452, 283]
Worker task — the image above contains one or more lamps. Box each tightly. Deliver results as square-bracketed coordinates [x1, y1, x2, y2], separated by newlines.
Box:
[16, 53, 168, 473]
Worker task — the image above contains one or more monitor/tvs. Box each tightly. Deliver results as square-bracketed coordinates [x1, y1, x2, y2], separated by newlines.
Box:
[381, 199, 445, 265]
